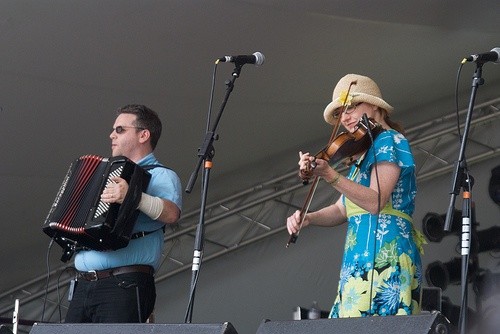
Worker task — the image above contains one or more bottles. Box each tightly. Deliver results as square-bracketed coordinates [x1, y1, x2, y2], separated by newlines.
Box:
[307, 300, 320, 320]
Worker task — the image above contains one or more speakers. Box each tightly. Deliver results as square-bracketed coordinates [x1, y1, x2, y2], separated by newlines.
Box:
[28, 321, 239, 334]
[257, 311, 458, 334]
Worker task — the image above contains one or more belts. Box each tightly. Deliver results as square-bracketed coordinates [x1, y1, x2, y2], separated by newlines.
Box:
[77, 265, 154, 282]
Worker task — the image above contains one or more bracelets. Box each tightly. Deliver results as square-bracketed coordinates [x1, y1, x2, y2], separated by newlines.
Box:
[329, 173, 342, 186]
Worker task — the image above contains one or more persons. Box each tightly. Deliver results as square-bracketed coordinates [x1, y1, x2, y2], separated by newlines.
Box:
[66, 104, 183, 323]
[286, 74, 426, 319]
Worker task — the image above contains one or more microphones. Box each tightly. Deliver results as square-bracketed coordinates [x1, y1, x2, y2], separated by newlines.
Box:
[466, 47, 500, 64]
[219, 51, 265, 65]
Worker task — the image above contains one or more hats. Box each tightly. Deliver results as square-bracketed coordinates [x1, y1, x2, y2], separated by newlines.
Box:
[323, 74, 393, 125]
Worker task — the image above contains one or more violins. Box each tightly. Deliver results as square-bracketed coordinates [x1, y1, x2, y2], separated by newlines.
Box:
[298, 117, 383, 186]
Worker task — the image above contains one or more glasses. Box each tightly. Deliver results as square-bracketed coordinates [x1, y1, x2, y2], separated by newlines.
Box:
[112, 126, 145, 133]
[332, 101, 363, 119]
